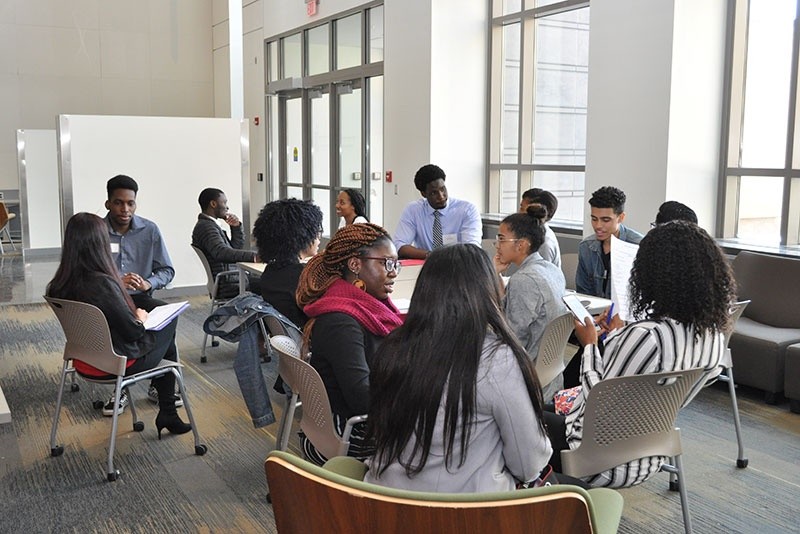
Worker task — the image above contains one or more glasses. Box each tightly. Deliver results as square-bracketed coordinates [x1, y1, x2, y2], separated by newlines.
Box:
[520, 204, 526, 210]
[650, 221, 657, 228]
[357, 257, 402, 274]
[495, 234, 531, 246]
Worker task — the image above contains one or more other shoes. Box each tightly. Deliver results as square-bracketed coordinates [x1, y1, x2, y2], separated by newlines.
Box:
[147, 387, 184, 406]
[102, 389, 130, 416]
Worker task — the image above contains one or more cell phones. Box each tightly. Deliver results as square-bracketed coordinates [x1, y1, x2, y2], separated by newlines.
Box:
[562, 294, 601, 332]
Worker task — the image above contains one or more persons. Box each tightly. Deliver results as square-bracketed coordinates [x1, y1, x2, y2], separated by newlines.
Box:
[294, 223, 404, 468]
[492, 188, 566, 406]
[45, 212, 193, 440]
[563, 186, 645, 389]
[538, 221, 738, 489]
[392, 164, 483, 260]
[102, 174, 183, 416]
[363, 243, 591, 494]
[191, 188, 274, 356]
[655, 201, 698, 224]
[335, 188, 369, 226]
[252, 198, 323, 398]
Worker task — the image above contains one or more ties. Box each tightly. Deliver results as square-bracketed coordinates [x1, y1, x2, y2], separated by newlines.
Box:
[433, 211, 443, 248]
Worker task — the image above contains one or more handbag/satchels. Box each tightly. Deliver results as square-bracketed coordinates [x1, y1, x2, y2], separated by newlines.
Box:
[554, 385, 582, 415]
[517, 463, 560, 490]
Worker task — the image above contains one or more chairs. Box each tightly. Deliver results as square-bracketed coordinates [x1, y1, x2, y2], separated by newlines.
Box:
[561, 367, 704, 534]
[67, 273, 187, 410]
[480, 238, 509, 275]
[265, 450, 625, 534]
[44, 295, 209, 482]
[670, 299, 751, 491]
[0, 203, 18, 256]
[562, 254, 579, 288]
[191, 244, 273, 363]
[271, 334, 369, 460]
[257, 314, 312, 504]
[722, 250, 800, 414]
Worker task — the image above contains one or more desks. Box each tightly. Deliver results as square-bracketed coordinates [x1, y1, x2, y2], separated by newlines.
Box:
[232, 259, 612, 316]
[0, 199, 21, 242]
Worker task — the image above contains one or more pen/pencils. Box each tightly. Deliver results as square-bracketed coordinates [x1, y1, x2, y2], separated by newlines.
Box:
[602, 302, 614, 339]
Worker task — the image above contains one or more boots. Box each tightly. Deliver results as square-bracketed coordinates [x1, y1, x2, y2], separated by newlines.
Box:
[150, 378, 192, 440]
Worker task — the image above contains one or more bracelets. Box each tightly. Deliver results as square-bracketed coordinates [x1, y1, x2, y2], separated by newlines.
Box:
[425, 251, 431, 258]
